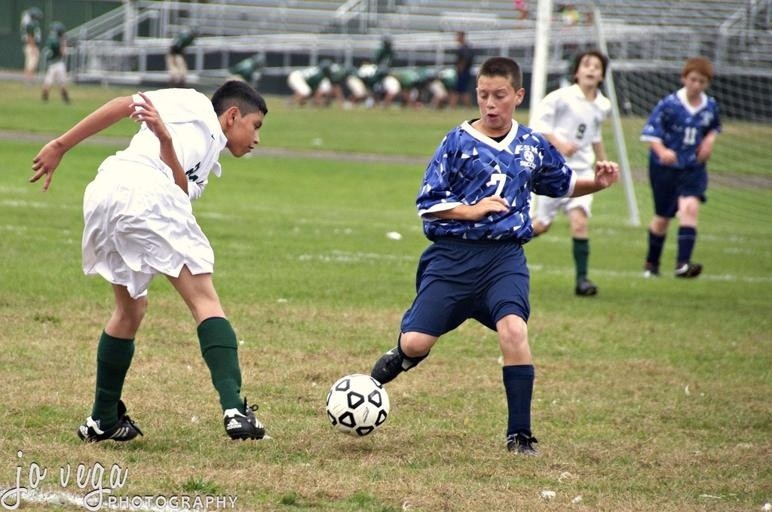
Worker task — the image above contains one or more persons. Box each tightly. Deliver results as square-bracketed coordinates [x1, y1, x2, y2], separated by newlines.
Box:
[164, 22, 201, 85]
[27, 81, 276, 442]
[514, 1, 595, 27]
[640, 57, 724, 281]
[19, 6, 44, 81]
[284, 29, 476, 113]
[369, 57, 619, 458]
[226, 51, 267, 85]
[529, 50, 613, 296]
[40, 20, 71, 104]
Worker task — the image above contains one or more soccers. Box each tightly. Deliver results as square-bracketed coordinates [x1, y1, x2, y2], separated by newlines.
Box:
[325, 373, 389, 436]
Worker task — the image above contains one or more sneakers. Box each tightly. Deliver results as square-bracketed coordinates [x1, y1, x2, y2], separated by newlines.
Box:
[675, 262, 703, 276]
[371, 348, 411, 383]
[506, 431, 536, 453]
[78, 415, 144, 443]
[575, 280, 597, 295]
[221, 407, 271, 440]
